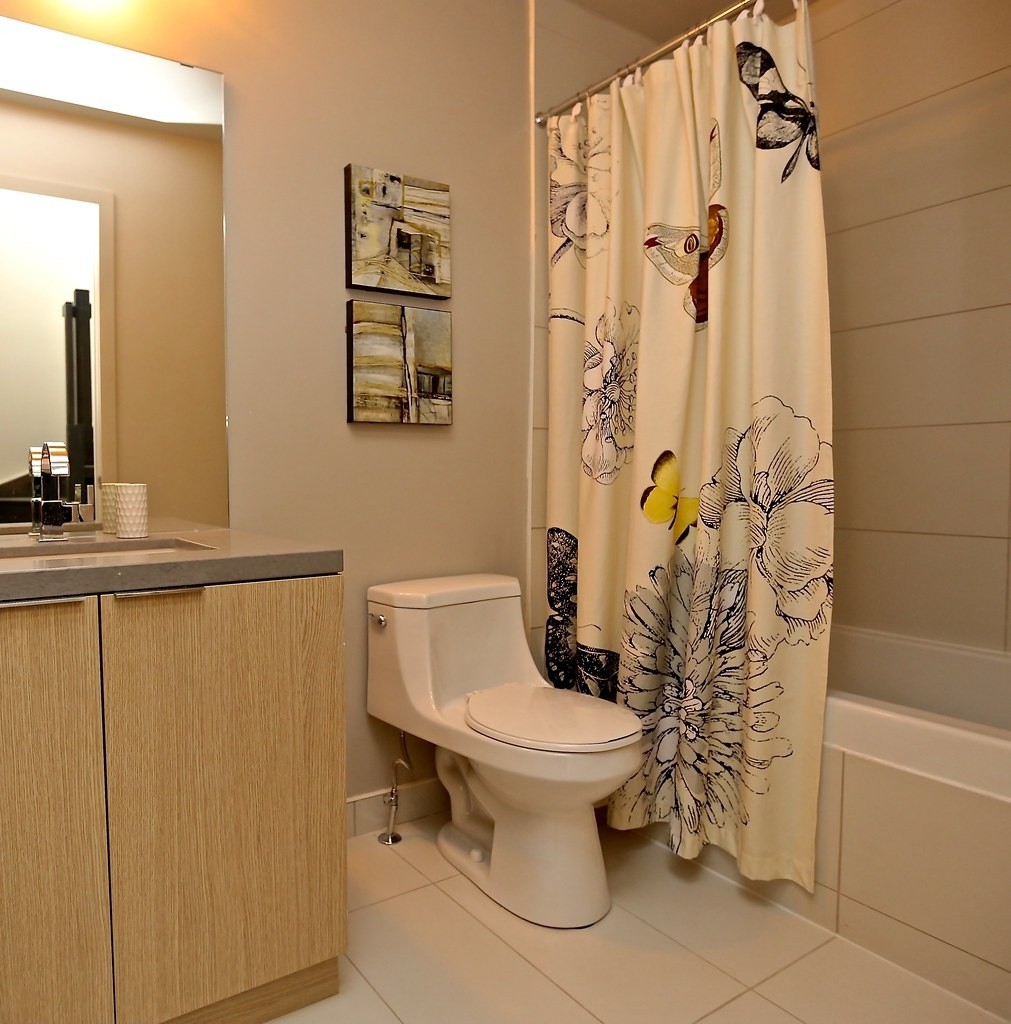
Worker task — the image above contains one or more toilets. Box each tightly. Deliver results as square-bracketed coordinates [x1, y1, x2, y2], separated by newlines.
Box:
[367, 571, 646, 930]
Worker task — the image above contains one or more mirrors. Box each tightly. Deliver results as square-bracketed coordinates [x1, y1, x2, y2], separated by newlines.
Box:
[0, 16, 231, 548]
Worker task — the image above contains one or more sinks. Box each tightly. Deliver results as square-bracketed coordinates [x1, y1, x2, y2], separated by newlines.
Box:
[0, 536, 222, 560]
[0, 523, 104, 536]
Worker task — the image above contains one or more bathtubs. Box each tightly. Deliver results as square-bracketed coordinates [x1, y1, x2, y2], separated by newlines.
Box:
[818, 624, 1011, 800]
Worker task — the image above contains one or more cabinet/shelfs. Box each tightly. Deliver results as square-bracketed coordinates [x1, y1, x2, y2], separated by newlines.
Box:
[0, 575, 347, 1024]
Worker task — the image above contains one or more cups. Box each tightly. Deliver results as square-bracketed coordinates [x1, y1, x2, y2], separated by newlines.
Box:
[100, 482, 117, 534]
[115, 483, 148, 538]
[74, 484, 94, 523]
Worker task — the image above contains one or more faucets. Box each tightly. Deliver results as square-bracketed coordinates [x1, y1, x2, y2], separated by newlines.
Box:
[39, 440, 84, 542]
[28, 446, 43, 536]
